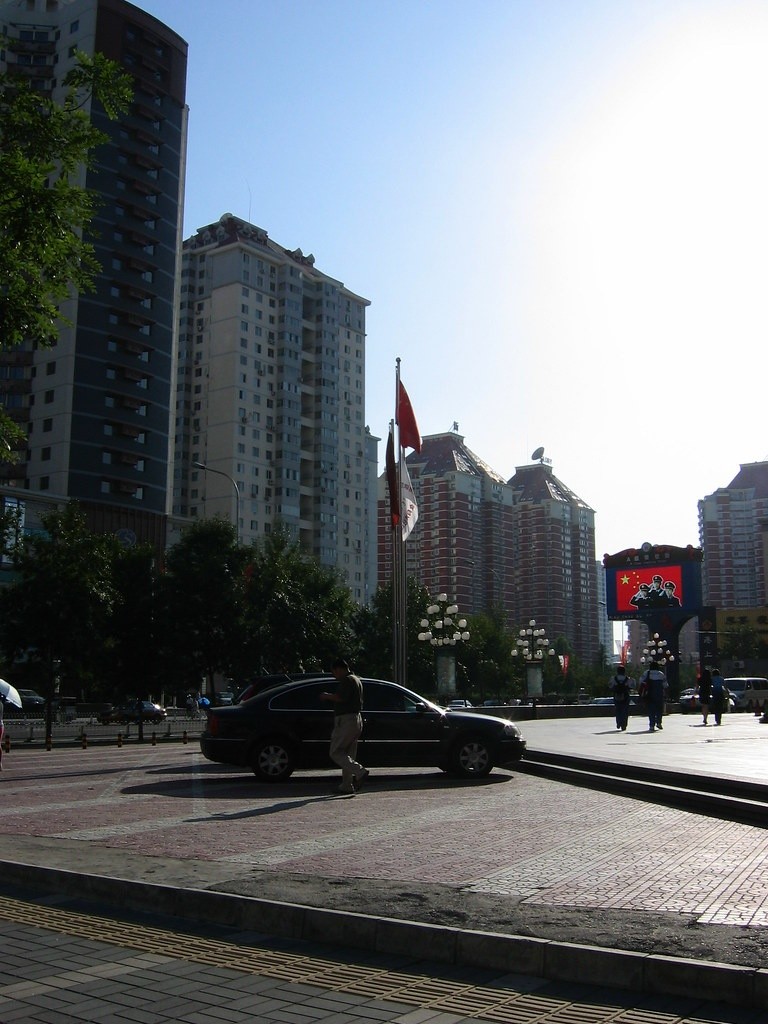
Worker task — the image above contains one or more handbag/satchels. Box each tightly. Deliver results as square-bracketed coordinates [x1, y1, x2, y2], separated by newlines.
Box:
[637, 682, 647, 697]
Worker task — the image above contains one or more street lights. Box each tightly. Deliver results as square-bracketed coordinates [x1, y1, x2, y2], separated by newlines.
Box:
[510, 619, 556, 697]
[193, 461, 241, 548]
[417, 593, 471, 703]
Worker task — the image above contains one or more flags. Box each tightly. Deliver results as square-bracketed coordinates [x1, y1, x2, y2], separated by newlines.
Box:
[386, 427, 400, 529]
[395, 370, 421, 454]
[400, 451, 418, 542]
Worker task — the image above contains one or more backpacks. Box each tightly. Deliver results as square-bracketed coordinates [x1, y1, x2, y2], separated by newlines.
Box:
[614, 676, 629, 703]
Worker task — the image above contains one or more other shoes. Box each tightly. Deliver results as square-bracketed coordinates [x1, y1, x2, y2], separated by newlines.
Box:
[656, 723, 663, 729]
[649, 727, 654, 731]
[355, 768, 370, 784]
[621, 727, 626, 731]
[617, 725, 620, 729]
[337, 784, 354, 793]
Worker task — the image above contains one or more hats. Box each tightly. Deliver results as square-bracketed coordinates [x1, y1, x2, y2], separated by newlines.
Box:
[640, 584, 651, 591]
[664, 582, 676, 592]
[652, 576, 663, 582]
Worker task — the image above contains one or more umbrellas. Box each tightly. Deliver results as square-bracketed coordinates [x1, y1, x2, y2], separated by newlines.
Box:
[198, 697, 210, 705]
[0, 679, 22, 709]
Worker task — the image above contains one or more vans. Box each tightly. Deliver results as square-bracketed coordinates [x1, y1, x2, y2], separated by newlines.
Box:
[578, 694, 591, 704]
[725, 677, 768, 713]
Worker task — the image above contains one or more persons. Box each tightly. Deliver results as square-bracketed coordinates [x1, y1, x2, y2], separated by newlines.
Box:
[0, 692, 4, 771]
[639, 661, 669, 730]
[697, 669, 713, 723]
[186, 690, 206, 719]
[608, 665, 636, 730]
[629, 575, 681, 610]
[322, 660, 369, 794]
[711, 669, 730, 725]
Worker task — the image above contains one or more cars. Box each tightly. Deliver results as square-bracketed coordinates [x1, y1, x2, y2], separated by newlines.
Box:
[97, 700, 167, 725]
[680, 685, 735, 713]
[16, 687, 46, 705]
[590, 696, 615, 704]
[217, 692, 234, 705]
[199, 677, 525, 779]
[447, 699, 472, 708]
[233, 674, 334, 704]
[482, 700, 507, 706]
